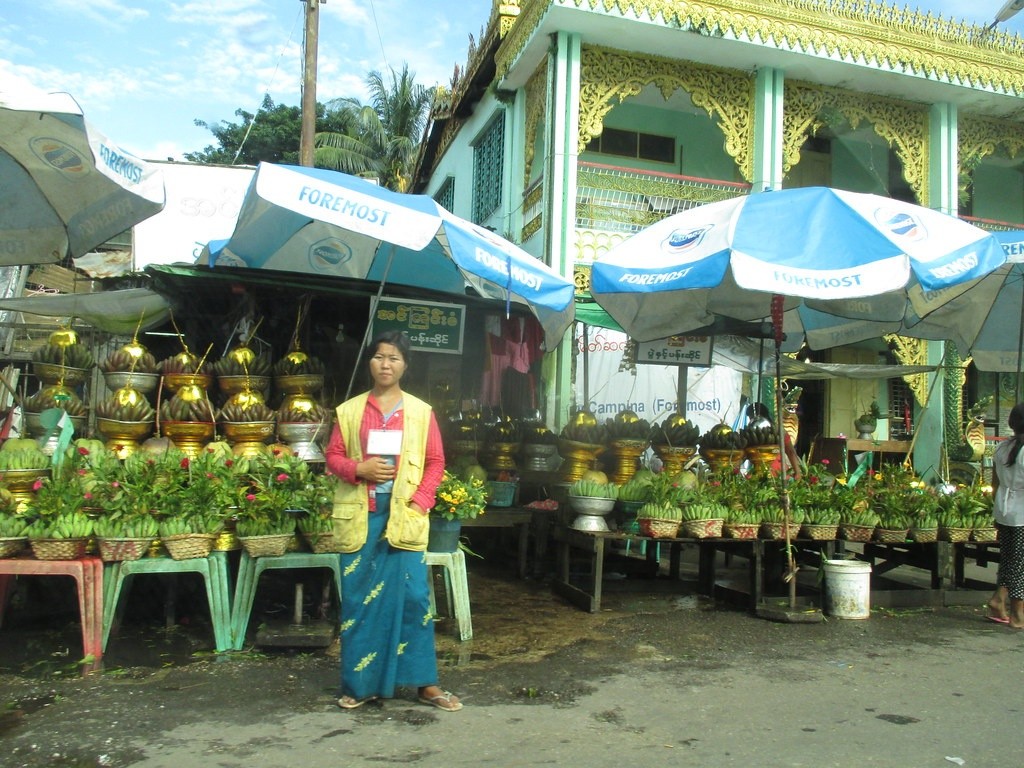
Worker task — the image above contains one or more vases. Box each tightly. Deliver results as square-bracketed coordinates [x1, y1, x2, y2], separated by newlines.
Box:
[428, 516, 461, 552]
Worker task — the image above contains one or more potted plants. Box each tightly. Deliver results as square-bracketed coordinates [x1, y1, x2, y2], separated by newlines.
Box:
[982, 446, 996, 467]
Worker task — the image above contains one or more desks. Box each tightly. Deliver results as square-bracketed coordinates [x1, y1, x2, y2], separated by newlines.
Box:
[460, 506, 1000, 614]
[0, 550, 343, 664]
[486, 466, 564, 569]
[580, 197, 648, 229]
[425, 549, 472, 641]
[819, 437, 914, 475]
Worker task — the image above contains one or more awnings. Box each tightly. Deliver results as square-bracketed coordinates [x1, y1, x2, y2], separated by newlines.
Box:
[710, 336, 967, 380]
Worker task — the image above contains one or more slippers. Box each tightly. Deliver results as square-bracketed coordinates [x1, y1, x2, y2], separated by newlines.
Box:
[984, 614, 1010, 623]
[419, 692, 463, 711]
[338, 695, 379, 708]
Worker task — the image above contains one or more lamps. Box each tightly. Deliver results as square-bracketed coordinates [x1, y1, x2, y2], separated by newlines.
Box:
[979, 0, 1024, 37]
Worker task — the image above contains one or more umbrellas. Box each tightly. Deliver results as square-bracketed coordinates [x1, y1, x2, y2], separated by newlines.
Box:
[0, 93, 167, 266]
[194, 159, 575, 403]
[572, 186, 1024, 610]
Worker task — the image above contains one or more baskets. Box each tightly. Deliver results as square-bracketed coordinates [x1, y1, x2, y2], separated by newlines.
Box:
[237, 533, 295, 558]
[30, 537, 88, 560]
[839, 522, 875, 541]
[638, 517, 682, 538]
[724, 525, 761, 539]
[161, 534, 219, 560]
[876, 529, 910, 542]
[95, 537, 159, 561]
[0, 537, 28, 558]
[761, 522, 801, 539]
[972, 528, 999, 541]
[910, 528, 939, 542]
[683, 518, 724, 538]
[303, 533, 337, 554]
[941, 528, 972, 542]
[801, 525, 839, 540]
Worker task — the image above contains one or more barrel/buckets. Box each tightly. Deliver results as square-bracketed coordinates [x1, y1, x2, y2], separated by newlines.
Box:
[823, 559, 872, 619]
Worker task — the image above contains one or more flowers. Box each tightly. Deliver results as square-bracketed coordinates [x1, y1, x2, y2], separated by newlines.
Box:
[434, 470, 490, 520]
[647, 455, 995, 509]
[0, 443, 340, 520]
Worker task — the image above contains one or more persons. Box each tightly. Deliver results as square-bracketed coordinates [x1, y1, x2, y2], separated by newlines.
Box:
[986, 403, 1024, 628]
[733, 402, 801, 483]
[326, 330, 466, 712]
